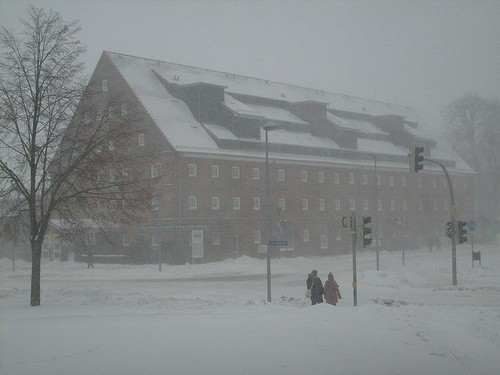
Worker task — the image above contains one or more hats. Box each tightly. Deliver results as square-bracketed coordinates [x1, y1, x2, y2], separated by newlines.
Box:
[328, 272, 333, 280]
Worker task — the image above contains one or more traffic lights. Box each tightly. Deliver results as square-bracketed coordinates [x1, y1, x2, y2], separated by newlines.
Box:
[414, 146, 424, 172]
[446, 221, 455, 238]
[458, 220, 467, 244]
[362, 215, 373, 248]
[343, 216, 353, 227]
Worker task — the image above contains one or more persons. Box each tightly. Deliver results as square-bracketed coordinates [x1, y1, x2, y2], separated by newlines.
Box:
[307, 270, 324, 305]
[87, 251, 93, 268]
[324, 272, 342, 306]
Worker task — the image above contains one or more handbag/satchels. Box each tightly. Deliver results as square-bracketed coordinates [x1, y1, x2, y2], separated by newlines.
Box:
[305, 289, 312, 297]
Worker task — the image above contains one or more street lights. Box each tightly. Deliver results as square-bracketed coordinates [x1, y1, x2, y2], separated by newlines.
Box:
[261, 120, 280, 304]
[364, 151, 380, 270]
